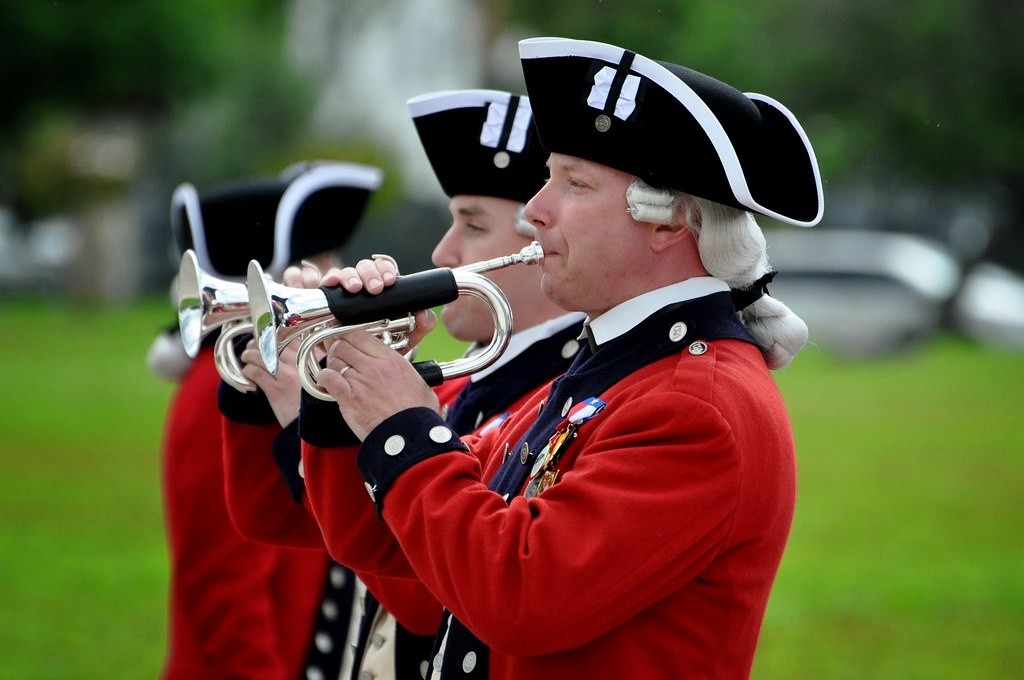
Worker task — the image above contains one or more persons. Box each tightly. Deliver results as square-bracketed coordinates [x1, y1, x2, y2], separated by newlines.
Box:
[215, 87, 592, 680]
[146, 158, 382, 680]
[299, 35, 826, 680]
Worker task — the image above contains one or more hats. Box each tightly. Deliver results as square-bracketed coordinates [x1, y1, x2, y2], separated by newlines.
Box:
[163, 156, 386, 275]
[405, 80, 553, 205]
[511, 30, 831, 232]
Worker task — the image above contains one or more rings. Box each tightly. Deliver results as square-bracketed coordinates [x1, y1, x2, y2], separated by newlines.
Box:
[338, 364, 352, 376]
